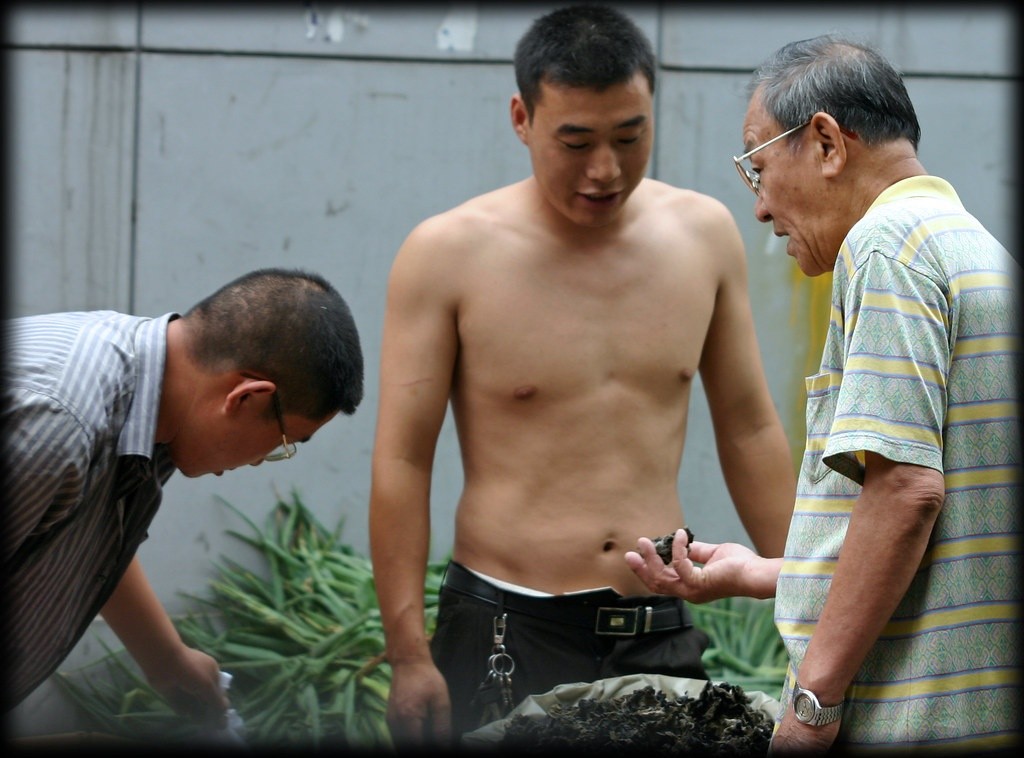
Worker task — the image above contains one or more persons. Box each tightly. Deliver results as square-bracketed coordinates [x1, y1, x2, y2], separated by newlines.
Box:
[370, 8, 793, 741]
[6, 269, 364, 730]
[626, 33, 1020, 749]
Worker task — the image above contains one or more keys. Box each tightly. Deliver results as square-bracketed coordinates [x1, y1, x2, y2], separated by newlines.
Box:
[478, 674, 515, 726]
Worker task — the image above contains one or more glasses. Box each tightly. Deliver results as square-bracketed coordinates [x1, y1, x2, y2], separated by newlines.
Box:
[732, 120, 861, 191]
[242, 372, 298, 462]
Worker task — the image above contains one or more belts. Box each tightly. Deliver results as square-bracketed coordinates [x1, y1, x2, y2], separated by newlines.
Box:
[440, 562, 694, 637]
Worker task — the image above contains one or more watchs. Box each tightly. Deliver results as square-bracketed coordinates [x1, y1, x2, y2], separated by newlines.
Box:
[793, 681, 844, 726]
